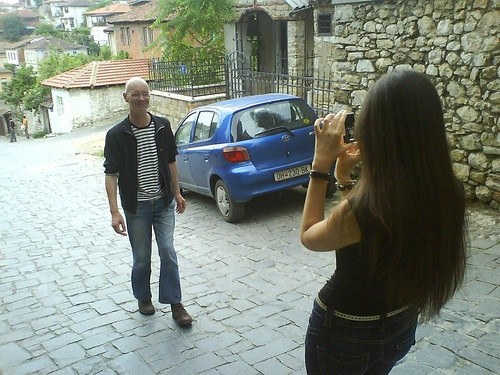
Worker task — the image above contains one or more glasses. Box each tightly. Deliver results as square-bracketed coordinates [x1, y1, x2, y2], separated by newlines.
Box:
[127, 92, 152, 99]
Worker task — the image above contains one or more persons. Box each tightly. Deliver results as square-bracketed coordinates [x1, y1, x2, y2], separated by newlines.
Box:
[300, 69, 468, 375]
[9, 117, 17, 142]
[22, 116, 30, 139]
[103, 77, 193, 328]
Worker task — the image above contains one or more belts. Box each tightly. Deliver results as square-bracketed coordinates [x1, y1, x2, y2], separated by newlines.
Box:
[314, 295, 419, 327]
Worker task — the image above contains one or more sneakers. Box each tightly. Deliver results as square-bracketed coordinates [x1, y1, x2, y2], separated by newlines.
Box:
[138, 299, 155, 315]
[171, 302, 193, 325]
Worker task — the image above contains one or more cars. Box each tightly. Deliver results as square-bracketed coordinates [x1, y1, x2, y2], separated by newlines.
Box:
[173, 93, 336, 223]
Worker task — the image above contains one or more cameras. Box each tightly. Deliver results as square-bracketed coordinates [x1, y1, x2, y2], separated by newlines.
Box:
[343, 112, 356, 143]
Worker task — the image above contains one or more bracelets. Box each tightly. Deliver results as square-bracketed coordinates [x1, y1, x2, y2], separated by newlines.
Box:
[335, 180, 355, 191]
[309, 169, 333, 182]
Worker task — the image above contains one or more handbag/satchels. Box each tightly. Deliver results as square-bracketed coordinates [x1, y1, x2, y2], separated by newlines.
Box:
[21, 124, 26, 131]
